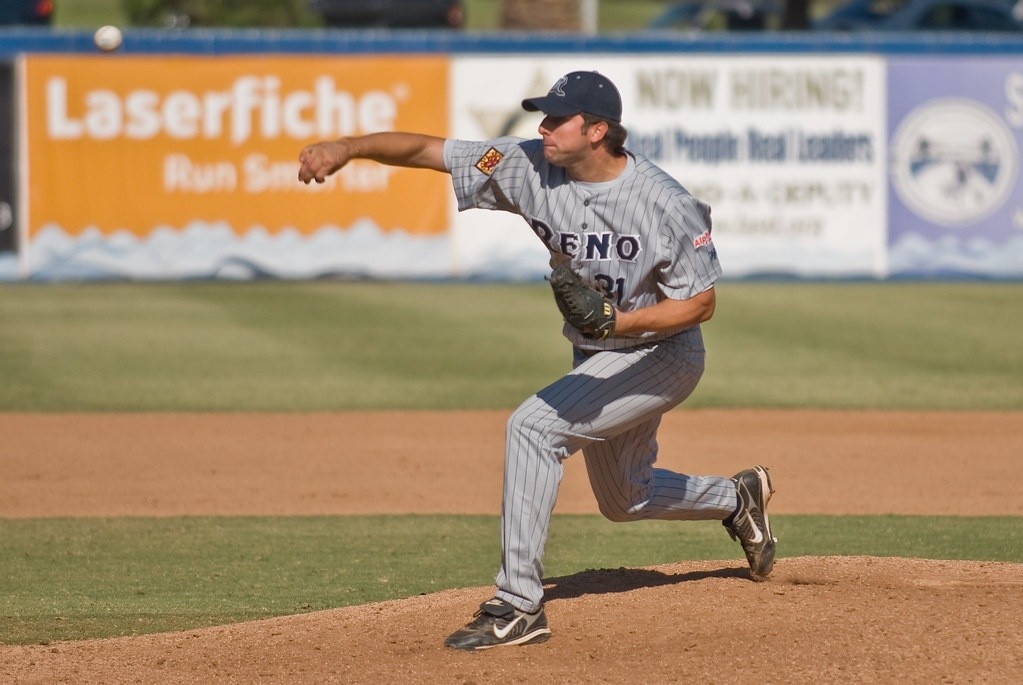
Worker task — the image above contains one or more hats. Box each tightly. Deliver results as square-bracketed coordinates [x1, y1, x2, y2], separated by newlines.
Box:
[522, 69, 623, 123]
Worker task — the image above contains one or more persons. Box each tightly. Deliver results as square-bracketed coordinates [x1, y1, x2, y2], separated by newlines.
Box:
[298, 72, 776, 650]
[314, 0, 460, 32]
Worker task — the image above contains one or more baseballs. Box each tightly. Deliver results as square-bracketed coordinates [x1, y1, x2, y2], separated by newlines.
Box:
[94, 25, 123, 51]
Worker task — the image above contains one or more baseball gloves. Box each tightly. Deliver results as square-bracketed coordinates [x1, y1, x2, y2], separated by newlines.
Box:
[542, 252, 616, 341]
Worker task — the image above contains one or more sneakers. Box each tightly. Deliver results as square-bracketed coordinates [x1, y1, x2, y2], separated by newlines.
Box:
[722, 464, 778, 582]
[444, 597, 551, 651]
